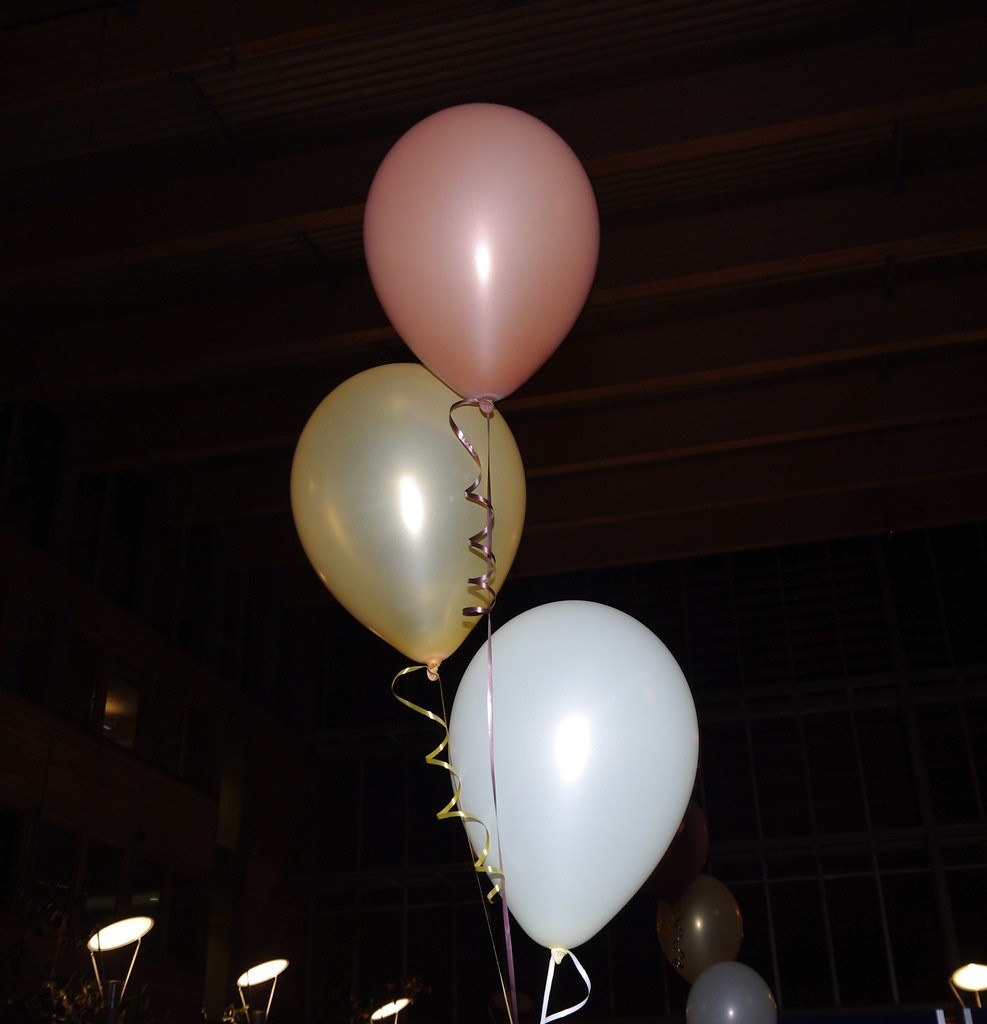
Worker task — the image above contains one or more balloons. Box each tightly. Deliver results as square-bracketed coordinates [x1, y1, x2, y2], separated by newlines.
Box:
[654, 875, 743, 982]
[687, 961, 778, 1024]
[447, 600, 701, 966]
[363, 103, 600, 418]
[288, 362, 527, 682]
